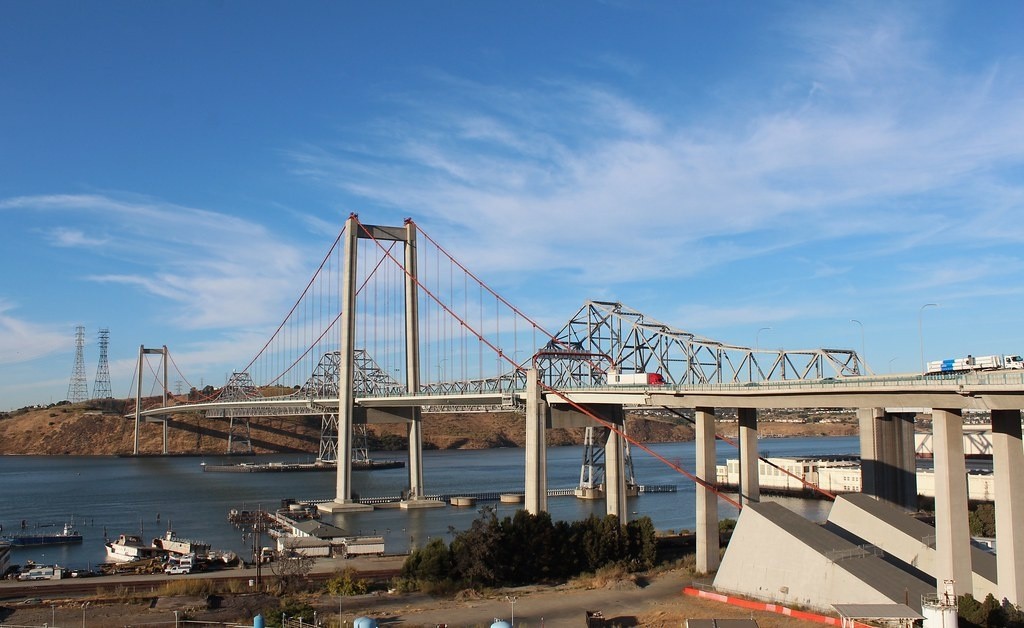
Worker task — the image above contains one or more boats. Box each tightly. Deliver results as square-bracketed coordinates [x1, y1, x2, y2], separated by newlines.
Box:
[1, 523, 83, 548]
[223, 551, 236, 563]
[152, 519, 211, 556]
[102, 519, 159, 565]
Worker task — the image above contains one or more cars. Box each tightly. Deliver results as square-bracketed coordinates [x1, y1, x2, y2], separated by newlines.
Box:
[209, 552, 215, 558]
[743, 382, 761, 388]
[819, 376, 842, 384]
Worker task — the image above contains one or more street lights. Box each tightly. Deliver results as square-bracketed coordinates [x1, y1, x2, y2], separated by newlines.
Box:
[505, 595, 517, 625]
[280, 611, 321, 628]
[172, 609, 179, 628]
[754, 327, 770, 383]
[79, 602, 89, 628]
[919, 303, 937, 375]
[850, 317, 868, 375]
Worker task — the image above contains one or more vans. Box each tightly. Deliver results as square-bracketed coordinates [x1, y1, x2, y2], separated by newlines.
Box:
[71, 569, 88, 578]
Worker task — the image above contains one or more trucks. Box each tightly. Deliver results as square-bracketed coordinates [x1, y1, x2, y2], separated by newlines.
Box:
[180, 553, 196, 568]
[607, 372, 671, 386]
[926, 354, 1024, 372]
[18, 568, 53, 580]
[197, 555, 212, 560]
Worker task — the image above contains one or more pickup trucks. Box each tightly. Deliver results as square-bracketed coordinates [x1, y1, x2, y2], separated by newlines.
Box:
[165, 567, 190, 575]
